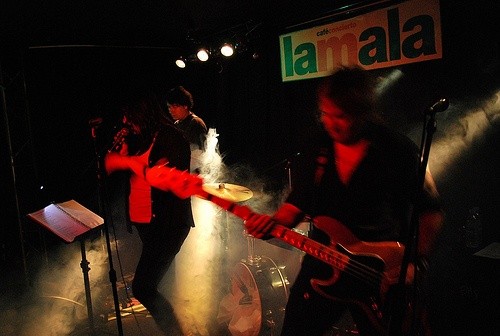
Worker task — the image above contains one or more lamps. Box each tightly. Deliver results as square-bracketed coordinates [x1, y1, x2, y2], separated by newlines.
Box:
[174, 33, 238, 69]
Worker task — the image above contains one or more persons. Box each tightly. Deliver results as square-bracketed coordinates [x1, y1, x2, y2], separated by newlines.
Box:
[113, 98, 195, 336]
[166, 87, 208, 175]
[243, 65, 444, 336]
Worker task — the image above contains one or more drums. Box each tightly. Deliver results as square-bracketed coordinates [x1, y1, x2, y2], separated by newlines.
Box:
[225, 255, 290, 336]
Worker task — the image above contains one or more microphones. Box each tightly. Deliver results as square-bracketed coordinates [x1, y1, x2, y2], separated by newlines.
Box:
[87, 118, 105, 128]
[426, 98, 449, 116]
[108, 130, 128, 153]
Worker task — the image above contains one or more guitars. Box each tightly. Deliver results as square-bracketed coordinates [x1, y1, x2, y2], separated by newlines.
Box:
[141, 160, 417, 333]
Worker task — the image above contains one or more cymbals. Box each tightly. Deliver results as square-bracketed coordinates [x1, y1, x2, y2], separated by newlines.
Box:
[201, 182, 254, 203]
